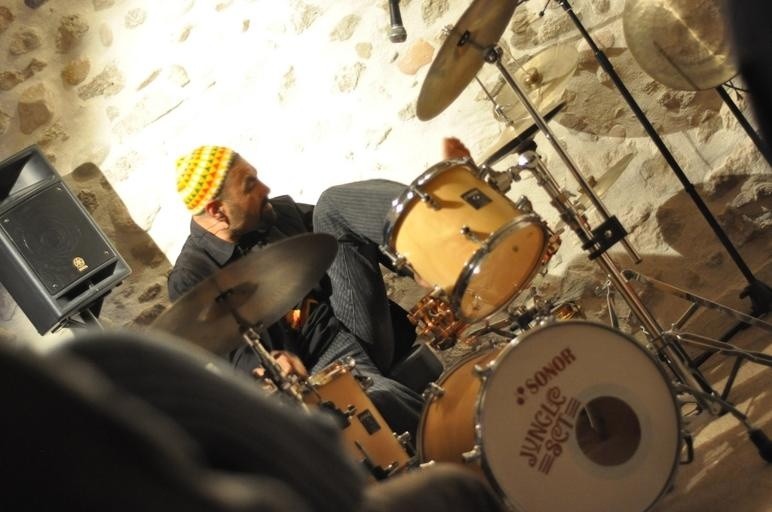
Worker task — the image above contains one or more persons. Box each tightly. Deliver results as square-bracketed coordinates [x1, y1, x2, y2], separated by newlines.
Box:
[167, 145, 427, 464]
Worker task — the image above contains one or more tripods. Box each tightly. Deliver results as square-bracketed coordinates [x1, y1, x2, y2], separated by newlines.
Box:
[516, 143, 772, 465]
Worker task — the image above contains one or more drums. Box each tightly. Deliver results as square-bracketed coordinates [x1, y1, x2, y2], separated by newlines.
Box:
[419, 319, 684, 512]
[381, 154, 551, 318]
[271, 359, 413, 477]
[517, 301, 588, 337]
[405, 285, 467, 345]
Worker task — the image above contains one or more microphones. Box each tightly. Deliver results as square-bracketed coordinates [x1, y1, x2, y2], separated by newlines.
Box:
[387, 0, 407, 43]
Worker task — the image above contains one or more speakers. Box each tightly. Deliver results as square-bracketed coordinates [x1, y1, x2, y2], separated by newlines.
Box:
[0, 144, 132, 336]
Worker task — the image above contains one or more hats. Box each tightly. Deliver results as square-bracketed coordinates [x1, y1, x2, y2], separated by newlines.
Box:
[174, 144, 238, 216]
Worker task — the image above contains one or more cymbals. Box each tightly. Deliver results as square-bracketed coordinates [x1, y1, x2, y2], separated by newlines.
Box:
[624, 1, 740, 88]
[148, 233, 337, 356]
[475, 100, 569, 170]
[556, 152, 637, 227]
[415, 0, 519, 120]
